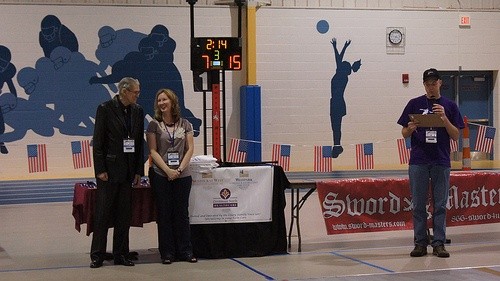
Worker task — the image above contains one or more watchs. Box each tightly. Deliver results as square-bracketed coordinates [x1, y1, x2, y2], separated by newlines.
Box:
[178, 168, 182, 173]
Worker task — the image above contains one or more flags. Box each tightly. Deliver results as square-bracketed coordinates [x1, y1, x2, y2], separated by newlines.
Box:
[27, 144, 47, 173]
[70, 141, 91, 169]
[313, 146, 333, 173]
[449, 136, 459, 153]
[228, 138, 249, 163]
[397, 136, 412, 164]
[474, 126, 496, 153]
[271, 144, 291, 172]
[355, 143, 374, 171]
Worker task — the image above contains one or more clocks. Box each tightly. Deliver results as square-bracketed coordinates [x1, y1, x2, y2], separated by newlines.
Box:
[389, 29, 402, 45]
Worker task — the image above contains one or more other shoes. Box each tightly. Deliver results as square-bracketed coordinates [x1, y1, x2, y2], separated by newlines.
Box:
[113, 255, 134, 266]
[90, 259, 103, 268]
[190, 255, 197, 262]
[162, 259, 171, 264]
[433, 245, 449, 257]
[410, 245, 427, 257]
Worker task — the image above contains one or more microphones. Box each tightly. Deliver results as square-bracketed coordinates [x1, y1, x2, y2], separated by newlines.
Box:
[431, 95, 437, 106]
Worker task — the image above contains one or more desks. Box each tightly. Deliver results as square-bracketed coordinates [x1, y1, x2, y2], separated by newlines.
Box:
[71, 180, 154, 237]
[188, 162, 292, 259]
[285, 172, 500, 253]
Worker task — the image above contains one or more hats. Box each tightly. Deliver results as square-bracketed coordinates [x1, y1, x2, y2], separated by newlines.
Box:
[424, 69, 439, 82]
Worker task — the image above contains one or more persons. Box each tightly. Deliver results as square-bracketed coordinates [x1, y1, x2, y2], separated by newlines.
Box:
[89, 77, 147, 268]
[397, 68, 464, 257]
[146, 88, 198, 264]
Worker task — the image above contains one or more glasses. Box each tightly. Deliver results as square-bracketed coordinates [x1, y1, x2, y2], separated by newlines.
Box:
[127, 89, 141, 95]
